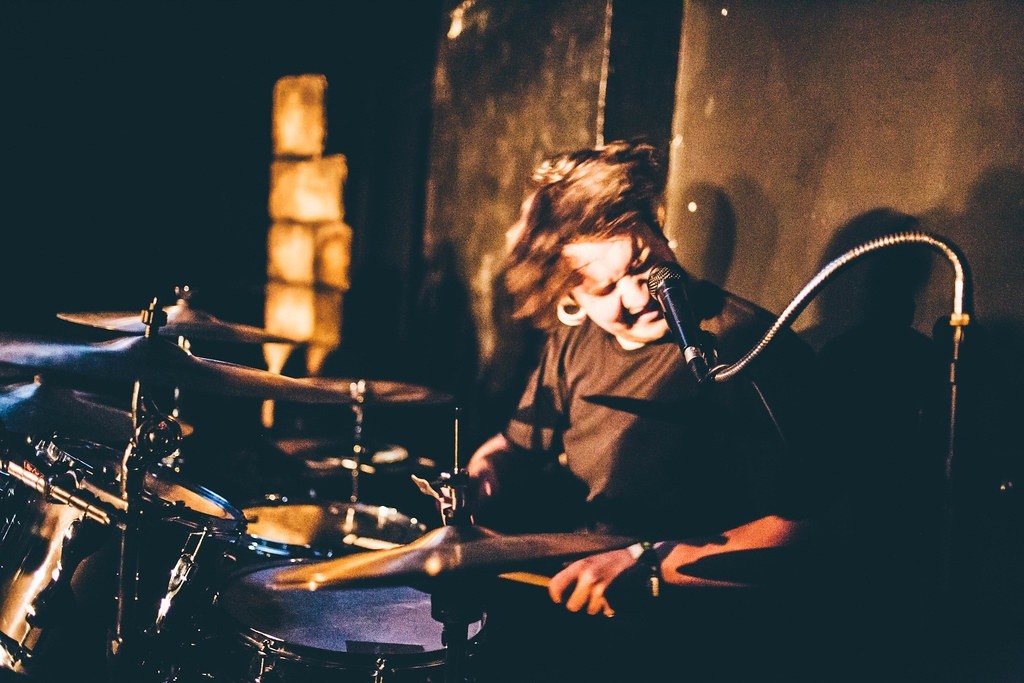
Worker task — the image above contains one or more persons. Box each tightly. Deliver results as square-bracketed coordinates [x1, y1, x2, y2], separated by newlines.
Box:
[432, 139, 831, 619]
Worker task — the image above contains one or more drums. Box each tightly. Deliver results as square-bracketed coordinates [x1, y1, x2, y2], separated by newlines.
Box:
[218, 554, 490, 683]
[0, 429, 247, 678]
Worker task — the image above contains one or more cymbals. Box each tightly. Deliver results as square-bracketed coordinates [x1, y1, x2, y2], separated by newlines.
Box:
[52, 298, 309, 348]
[260, 516, 638, 596]
[243, 496, 421, 555]
[0, 329, 351, 409]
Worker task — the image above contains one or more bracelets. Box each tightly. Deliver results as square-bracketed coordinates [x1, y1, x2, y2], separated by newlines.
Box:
[624, 540, 664, 599]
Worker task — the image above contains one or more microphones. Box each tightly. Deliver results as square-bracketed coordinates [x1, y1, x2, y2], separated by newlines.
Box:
[648, 261, 710, 386]
